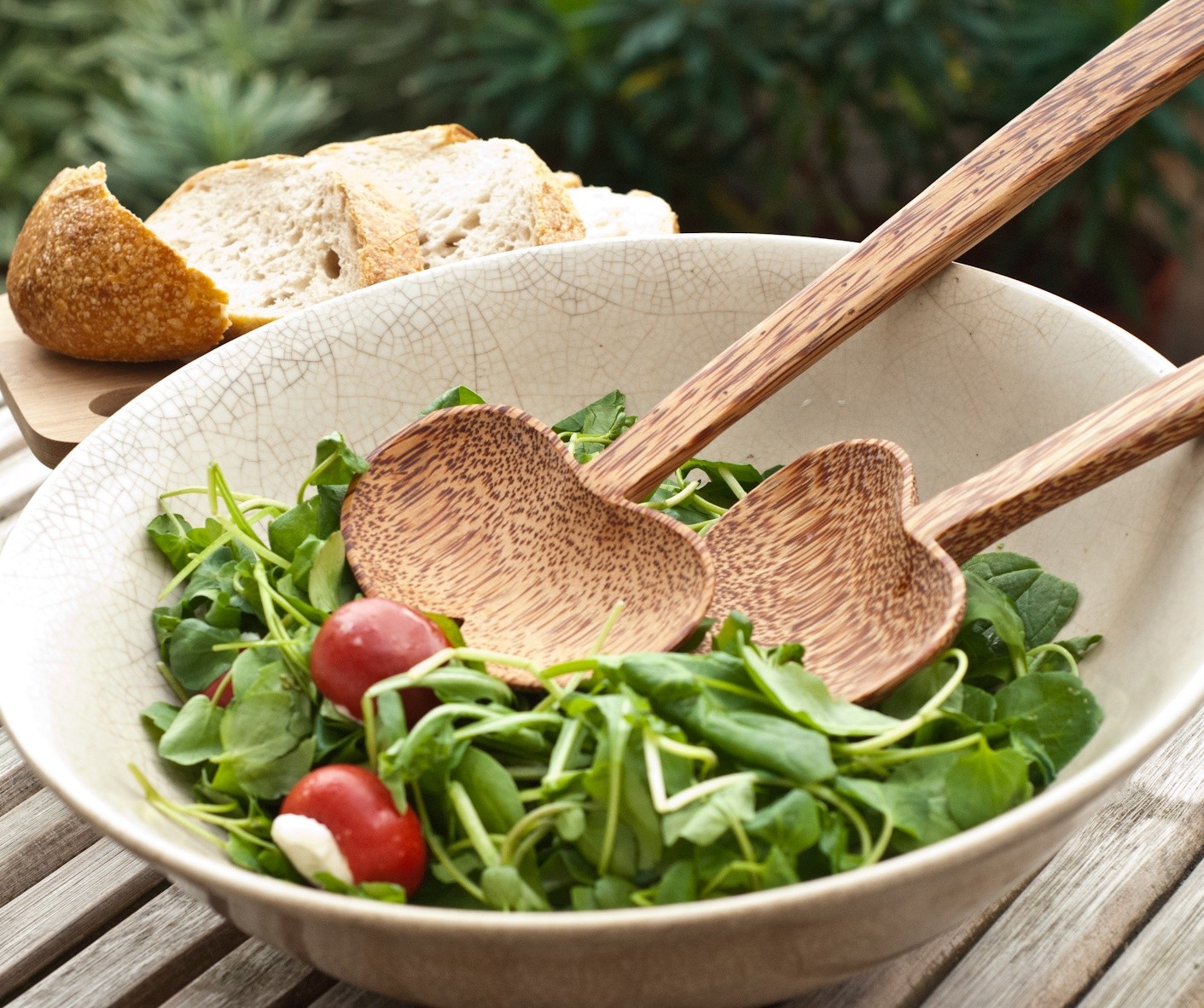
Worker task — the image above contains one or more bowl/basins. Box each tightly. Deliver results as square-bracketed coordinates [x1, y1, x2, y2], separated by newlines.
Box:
[1, 238, 1204, 1007]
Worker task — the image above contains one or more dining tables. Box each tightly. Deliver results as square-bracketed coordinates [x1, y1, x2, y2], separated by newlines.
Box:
[0, 395, 1204, 1008]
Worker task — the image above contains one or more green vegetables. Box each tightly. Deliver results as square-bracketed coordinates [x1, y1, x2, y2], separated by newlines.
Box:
[146, 391, 1108, 913]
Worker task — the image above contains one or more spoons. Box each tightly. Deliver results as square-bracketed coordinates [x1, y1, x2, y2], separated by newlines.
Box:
[339, 0, 1204, 697]
[699, 338, 1204, 707]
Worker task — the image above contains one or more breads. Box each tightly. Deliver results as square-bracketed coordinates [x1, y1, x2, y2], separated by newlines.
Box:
[2, 119, 682, 365]
